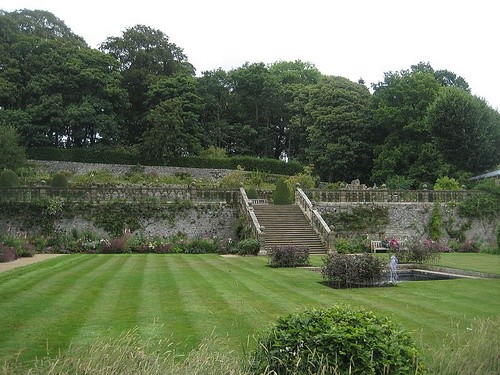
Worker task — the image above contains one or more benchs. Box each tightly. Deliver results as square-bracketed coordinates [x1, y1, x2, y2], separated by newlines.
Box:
[370, 239, 408, 254]
